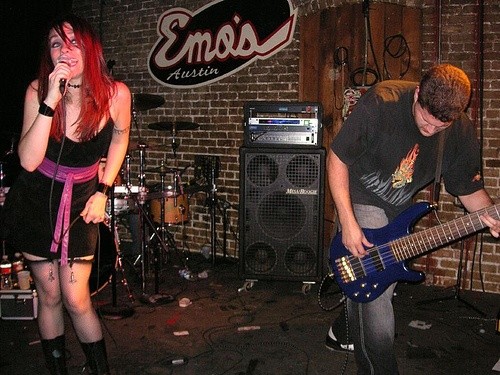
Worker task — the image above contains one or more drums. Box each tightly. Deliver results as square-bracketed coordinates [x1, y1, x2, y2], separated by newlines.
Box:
[149, 183, 190, 226]
[105, 186, 148, 212]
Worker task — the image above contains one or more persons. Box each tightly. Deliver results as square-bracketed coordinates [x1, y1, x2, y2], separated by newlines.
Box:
[0, 14, 131, 375]
[326, 65, 500, 375]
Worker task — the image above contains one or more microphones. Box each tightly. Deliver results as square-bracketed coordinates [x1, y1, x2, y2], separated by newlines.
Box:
[57, 54, 70, 92]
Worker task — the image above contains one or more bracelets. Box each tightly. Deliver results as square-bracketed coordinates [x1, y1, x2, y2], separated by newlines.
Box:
[38, 102, 54, 117]
[97, 183, 112, 196]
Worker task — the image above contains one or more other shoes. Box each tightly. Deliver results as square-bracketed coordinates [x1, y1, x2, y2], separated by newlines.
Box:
[324, 325, 356, 354]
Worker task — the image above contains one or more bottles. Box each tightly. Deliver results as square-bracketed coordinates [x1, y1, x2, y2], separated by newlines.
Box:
[0, 256, 12, 290]
[178, 269, 198, 281]
[12, 252, 24, 287]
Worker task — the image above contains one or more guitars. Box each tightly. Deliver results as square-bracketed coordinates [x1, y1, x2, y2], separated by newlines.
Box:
[329, 201, 500, 303]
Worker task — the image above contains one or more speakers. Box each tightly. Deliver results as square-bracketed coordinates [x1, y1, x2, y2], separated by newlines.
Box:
[237, 146, 326, 282]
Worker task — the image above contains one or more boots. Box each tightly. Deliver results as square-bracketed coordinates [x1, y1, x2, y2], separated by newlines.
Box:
[41, 334, 68, 375]
[80, 337, 111, 375]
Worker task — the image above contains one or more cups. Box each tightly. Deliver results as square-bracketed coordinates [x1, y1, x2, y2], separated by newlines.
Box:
[17, 271, 31, 289]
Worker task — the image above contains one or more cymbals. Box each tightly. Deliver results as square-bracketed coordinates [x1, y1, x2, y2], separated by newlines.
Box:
[147, 121, 200, 130]
[130, 93, 165, 110]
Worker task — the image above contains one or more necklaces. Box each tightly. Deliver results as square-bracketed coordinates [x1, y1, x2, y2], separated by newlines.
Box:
[66, 83, 81, 88]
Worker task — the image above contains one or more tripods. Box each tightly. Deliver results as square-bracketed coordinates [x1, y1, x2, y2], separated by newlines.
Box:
[131, 140, 189, 274]
[414, 206, 488, 318]
